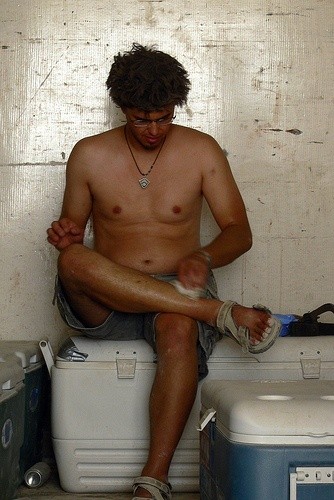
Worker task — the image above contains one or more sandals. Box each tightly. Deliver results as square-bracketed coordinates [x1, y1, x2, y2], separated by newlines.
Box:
[216, 300, 282, 354]
[131, 476, 172, 500]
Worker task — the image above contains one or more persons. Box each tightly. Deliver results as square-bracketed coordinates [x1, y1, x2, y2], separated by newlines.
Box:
[46, 43, 280, 500]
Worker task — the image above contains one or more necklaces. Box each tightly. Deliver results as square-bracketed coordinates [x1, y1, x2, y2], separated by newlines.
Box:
[123, 123, 167, 188]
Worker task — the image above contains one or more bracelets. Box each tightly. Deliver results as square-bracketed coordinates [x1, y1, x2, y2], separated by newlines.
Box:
[196, 248, 212, 270]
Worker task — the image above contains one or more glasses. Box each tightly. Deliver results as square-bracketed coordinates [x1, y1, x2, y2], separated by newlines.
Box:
[124, 110, 176, 128]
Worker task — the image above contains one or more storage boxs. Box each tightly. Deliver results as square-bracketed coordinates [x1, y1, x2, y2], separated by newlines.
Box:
[0, 336, 334, 500]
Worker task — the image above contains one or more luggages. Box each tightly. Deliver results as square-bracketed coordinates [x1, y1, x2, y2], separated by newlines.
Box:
[193, 378, 334, 500]
[38, 334, 334, 493]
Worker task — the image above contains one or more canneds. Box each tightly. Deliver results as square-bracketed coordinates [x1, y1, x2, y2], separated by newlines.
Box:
[22, 461, 52, 488]
